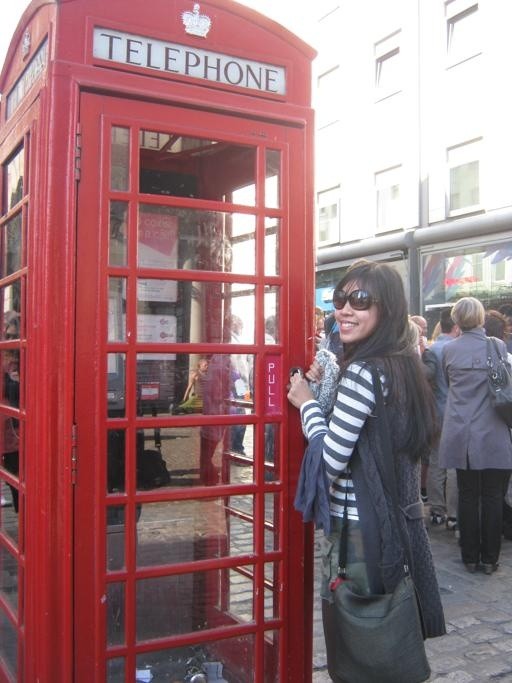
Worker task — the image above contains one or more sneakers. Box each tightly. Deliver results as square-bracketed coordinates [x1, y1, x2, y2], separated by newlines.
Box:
[483, 562, 499, 574]
[466, 561, 481, 572]
[430, 512, 445, 526]
[446, 517, 458, 531]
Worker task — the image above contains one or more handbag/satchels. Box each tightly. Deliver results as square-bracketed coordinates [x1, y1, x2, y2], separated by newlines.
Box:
[135, 445, 170, 490]
[325, 571, 432, 682]
[483, 336, 511, 428]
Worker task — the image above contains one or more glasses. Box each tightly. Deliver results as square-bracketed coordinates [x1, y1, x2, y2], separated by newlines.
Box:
[331, 289, 382, 312]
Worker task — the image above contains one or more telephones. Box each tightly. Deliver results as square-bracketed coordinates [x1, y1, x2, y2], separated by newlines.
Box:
[106, 407, 143, 534]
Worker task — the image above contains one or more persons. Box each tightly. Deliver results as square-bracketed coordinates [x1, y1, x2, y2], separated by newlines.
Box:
[104, 358, 146, 644]
[287, 262, 445, 682]
[1, 310, 20, 510]
[183, 259, 512, 575]
